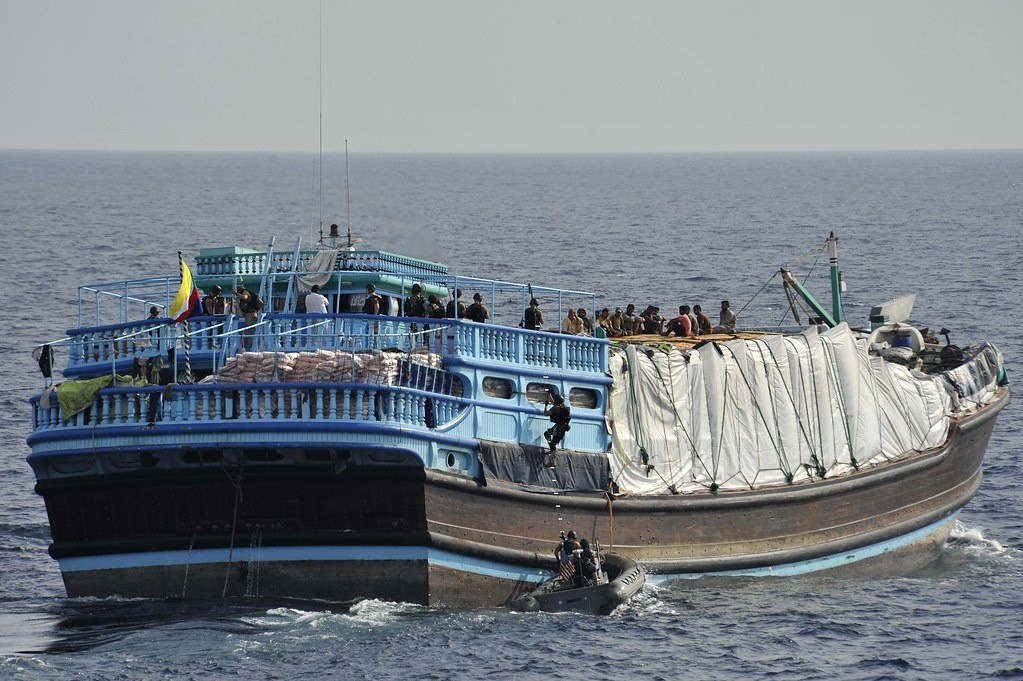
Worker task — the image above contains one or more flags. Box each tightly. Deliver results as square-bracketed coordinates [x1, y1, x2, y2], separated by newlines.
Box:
[168, 255, 204, 323]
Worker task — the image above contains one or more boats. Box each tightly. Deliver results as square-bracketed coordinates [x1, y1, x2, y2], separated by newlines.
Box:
[28, 233, 1010, 612]
[520, 551, 646, 616]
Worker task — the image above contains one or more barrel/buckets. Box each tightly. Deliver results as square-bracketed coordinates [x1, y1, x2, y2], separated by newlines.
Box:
[891, 334, 912, 347]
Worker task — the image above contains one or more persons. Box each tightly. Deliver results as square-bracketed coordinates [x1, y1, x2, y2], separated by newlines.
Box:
[147, 306, 162, 351]
[305, 284, 330, 331]
[446, 289, 465, 318]
[524, 299, 545, 331]
[234, 284, 260, 349]
[201, 284, 225, 348]
[561, 300, 737, 340]
[555, 531, 581, 571]
[544, 391, 571, 454]
[578, 538, 596, 565]
[404, 282, 428, 343]
[362, 282, 384, 348]
[467, 292, 490, 324]
[423, 294, 444, 346]
